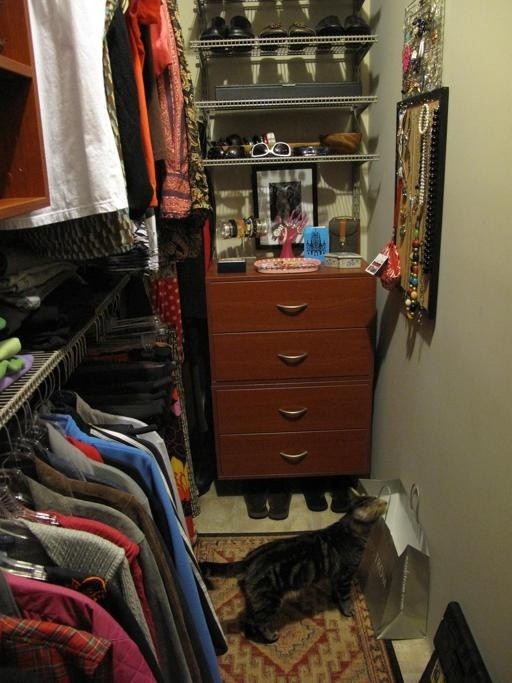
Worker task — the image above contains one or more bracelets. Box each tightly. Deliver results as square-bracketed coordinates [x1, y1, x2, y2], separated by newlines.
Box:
[219, 215, 270, 238]
[242, 131, 277, 146]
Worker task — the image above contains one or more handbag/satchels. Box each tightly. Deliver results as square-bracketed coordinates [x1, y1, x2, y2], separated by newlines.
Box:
[330, 216, 360, 255]
[356, 478, 432, 640]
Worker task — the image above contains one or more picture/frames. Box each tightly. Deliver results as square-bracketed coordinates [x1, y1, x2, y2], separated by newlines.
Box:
[249, 161, 319, 251]
[386, 85, 451, 322]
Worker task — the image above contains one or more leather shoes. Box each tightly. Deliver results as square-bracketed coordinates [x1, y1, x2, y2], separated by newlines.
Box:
[200, 15, 371, 53]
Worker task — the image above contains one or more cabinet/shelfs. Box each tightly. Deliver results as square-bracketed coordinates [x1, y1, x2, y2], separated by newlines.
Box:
[188, 0, 383, 168]
[202, 260, 380, 485]
[0, 0, 52, 222]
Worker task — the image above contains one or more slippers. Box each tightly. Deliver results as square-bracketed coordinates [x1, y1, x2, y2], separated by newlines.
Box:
[243, 481, 269, 518]
[268, 481, 292, 520]
[330, 477, 353, 512]
[304, 477, 328, 512]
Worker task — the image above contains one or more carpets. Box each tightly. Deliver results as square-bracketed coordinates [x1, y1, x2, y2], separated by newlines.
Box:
[185, 530, 408, 682]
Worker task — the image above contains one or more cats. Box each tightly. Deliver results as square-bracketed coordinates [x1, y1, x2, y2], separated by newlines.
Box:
[198, 486, 388, 643]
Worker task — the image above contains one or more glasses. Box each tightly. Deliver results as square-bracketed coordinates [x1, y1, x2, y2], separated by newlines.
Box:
[249, 141, 294, 158]
[207, 145, 247, 159]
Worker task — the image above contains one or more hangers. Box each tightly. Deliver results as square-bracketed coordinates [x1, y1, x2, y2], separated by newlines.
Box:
[1, 285, 129, 589]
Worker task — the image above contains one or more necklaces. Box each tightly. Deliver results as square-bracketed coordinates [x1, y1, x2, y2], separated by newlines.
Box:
[397, 104, 440, 329]
